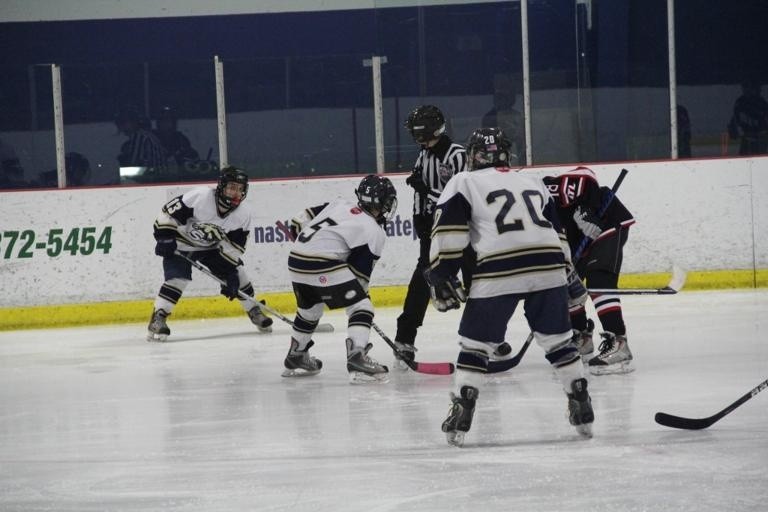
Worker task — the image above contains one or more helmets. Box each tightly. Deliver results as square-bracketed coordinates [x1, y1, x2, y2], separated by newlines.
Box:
[218, 165, 249, 208]
[63, 152, 91, 178]
[400, 103, 446, 147]
[154, 104, 177, 128]
[357, 175, 398, 224]
[466, 127, 513, 171]
[117, 107, 142, 132]
[491, 82, 517, 107]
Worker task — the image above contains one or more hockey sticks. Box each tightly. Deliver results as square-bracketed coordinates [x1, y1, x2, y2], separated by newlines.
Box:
[487, 170, 628, 374]
[654, 379, 768, 431]
[176, 249, 334, 333]
[370, 319, 455, 375]
[588, 264, 688, 296]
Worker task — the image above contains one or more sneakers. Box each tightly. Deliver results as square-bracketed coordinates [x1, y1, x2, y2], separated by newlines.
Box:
[348, 338, 384, 373]
[392, 341, 416, 364]
[566, 377, 595, 426]
[148, 309, 170, 334]
[570, 329, 596, 355]
[283, 337, 322, 373]
[589, 331, 634, 366]
[246, 304, 272, 329]
[441, 387, 478, 432]
[488, 342, 512, 362]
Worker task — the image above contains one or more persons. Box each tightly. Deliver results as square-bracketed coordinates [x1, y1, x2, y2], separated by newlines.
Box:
[149, 99, 218, 181]
[36, 151, 93, 189]
[476, 85, 527, 165]
[147, 166, 273, 342]
[424, 128, 594, 446]
[728, 75, 768, 156]
[395, 104, 512, 370]
[541, 165, 636, 374]
[112, 101, 179, 184]
[280, 174, 397, 386]
